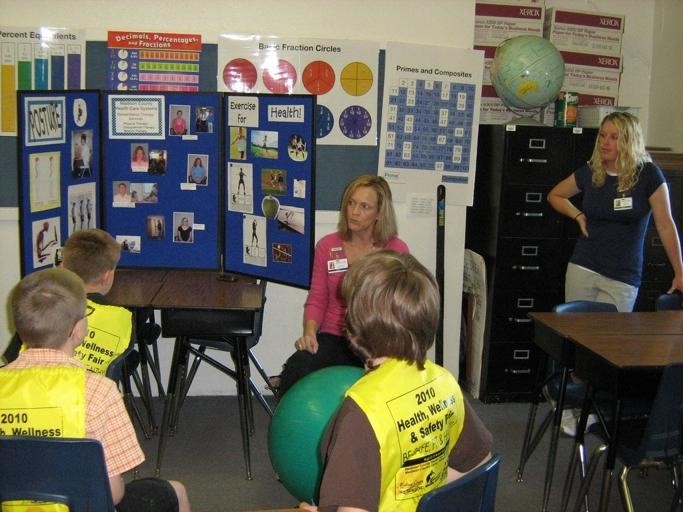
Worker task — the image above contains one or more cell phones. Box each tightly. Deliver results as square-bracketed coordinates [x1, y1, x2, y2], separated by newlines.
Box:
[215, 274, 240, 282]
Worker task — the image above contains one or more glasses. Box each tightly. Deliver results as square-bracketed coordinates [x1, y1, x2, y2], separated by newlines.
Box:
[69, 305, 94, 335]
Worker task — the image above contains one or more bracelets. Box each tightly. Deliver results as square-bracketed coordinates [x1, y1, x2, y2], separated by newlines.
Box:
[574, 212, 583, 220]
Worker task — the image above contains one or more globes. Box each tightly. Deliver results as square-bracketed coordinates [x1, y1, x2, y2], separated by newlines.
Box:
[490, 35, 565, 126]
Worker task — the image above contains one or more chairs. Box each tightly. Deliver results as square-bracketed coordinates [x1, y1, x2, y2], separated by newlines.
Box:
[1, 267, 278, 480]
[0, 436, 117, 512]
[409, 291, 681, 512]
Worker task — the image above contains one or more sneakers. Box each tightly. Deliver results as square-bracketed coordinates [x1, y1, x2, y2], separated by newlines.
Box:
[541, 385, 601, 436]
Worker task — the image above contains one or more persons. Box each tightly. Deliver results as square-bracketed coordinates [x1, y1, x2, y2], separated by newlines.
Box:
[299, 255, 494, 512]
[1, 267, 192, 511]
[276, 176, 409, 400]
[540, 110, 683, 436]
[1, 225, 139, 394]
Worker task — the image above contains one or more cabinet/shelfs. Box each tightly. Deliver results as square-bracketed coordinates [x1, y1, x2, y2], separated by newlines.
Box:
[465, 124, 681, 405]
